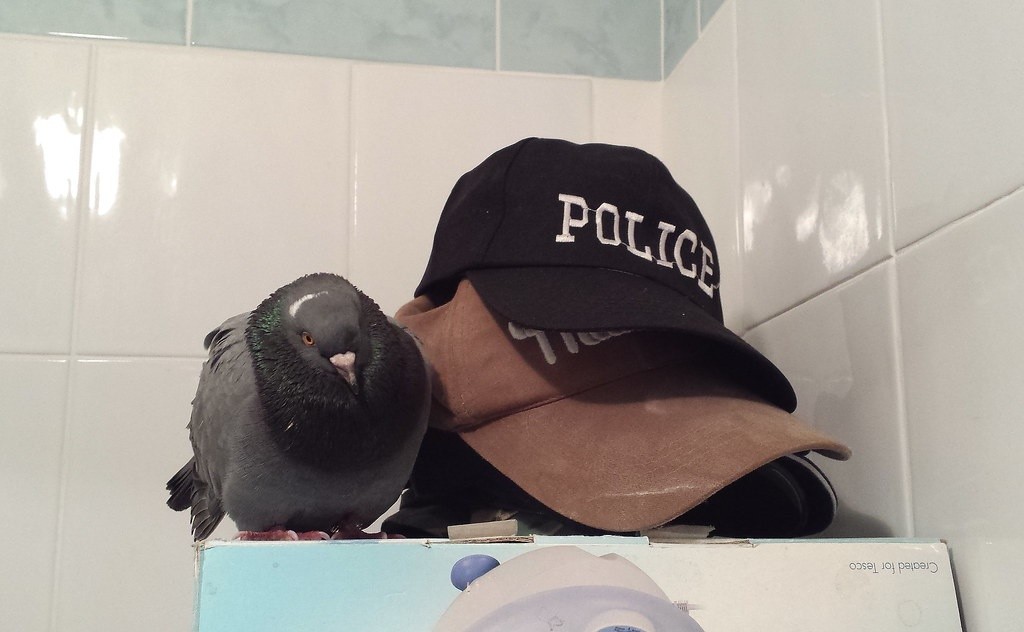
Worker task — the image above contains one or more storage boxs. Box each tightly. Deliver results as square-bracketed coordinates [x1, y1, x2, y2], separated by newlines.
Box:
[192, 520, 963, 632]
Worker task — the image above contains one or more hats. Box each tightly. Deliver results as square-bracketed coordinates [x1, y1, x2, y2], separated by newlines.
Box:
[397, 136, 851, 538]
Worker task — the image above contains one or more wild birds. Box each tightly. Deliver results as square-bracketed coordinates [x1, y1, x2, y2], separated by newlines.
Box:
[165, 274, 431, 543]
